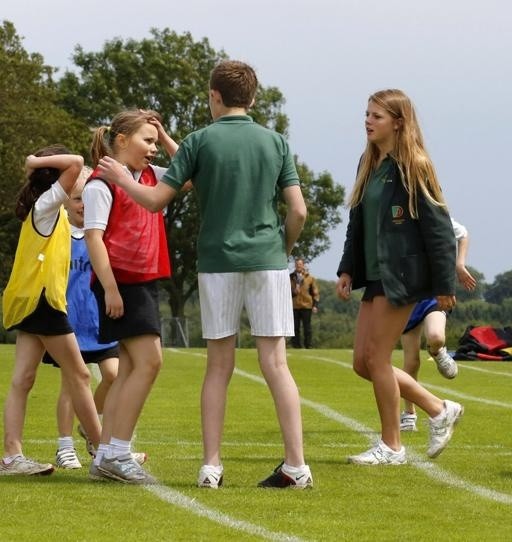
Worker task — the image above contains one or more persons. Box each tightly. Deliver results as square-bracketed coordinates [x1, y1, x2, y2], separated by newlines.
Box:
[398, 200, 475, 433]
[0, 144, 150, 481]
[287, 260, 321, 350]
[337, 89, 465, 470]
[94, 61, 315, 489]
[82, 110, 195, 484]
[54, 164, 139, 470]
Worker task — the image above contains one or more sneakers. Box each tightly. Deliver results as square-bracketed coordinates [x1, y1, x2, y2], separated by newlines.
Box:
[258, 461, 313, 489]
[56, 423, 156, 484]
[349, 439, 407, 465]
[0, 455, 54, 475]
[427, 343, 458, 379]
[399, 410, 417, 431]
[427, 399, 464, 458]
[197, 464, 224, 489]
